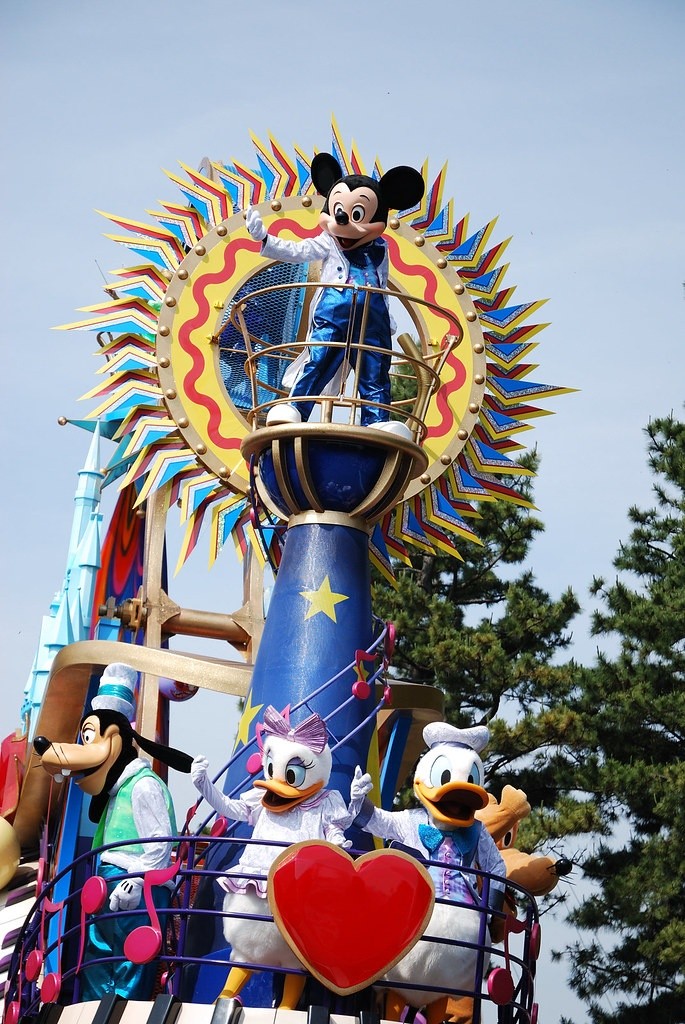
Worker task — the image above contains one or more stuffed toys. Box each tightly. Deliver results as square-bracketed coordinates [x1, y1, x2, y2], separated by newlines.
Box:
[191, 705, 353, 1009]
[242, 152, 425, 442]
[34, 662, 195, 1002]
[348, 722, 572, 1024]
